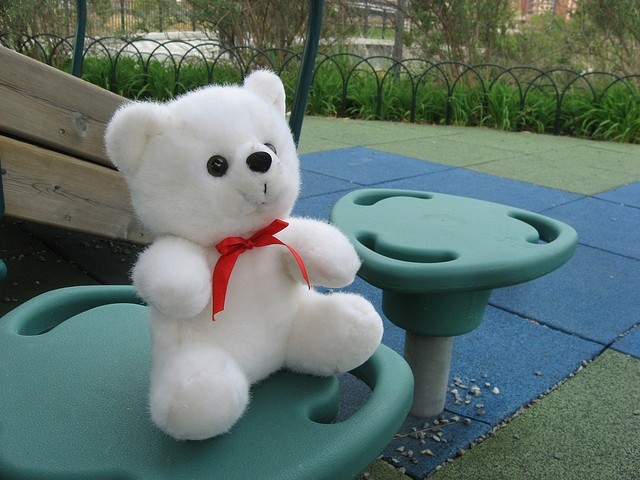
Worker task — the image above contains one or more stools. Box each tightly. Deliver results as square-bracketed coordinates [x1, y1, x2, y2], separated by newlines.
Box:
[327, 187, 578, 418]
[2, 281, 415, 478]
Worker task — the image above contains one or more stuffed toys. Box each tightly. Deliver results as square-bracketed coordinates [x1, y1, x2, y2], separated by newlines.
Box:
[102, 68, 385, 443]
